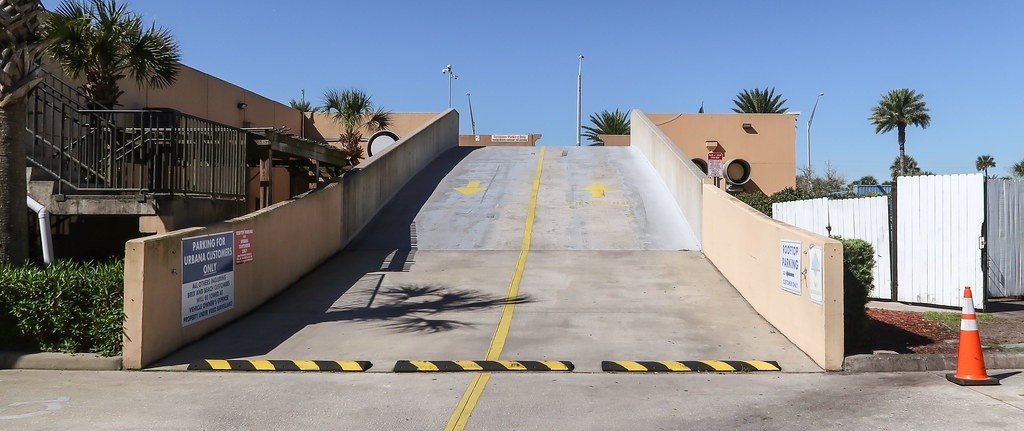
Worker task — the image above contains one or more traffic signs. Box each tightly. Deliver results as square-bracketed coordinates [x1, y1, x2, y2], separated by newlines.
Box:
[780, 239, 802, 296]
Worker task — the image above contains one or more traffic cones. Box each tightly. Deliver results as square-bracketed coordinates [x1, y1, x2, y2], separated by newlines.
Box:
[945, 286, 1000, 386]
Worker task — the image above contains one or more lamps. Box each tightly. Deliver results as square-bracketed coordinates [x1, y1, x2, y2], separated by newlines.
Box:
[743, 123, 751, 128]
[237, 102, 248, 109]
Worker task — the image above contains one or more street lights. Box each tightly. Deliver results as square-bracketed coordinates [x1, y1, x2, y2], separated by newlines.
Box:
[465, 91, 475, 134]
[576, 54, 585, 147]
[301, 89, 304, 139]
[441, 64, 458, 108]
[807, 92, 825, 180]
